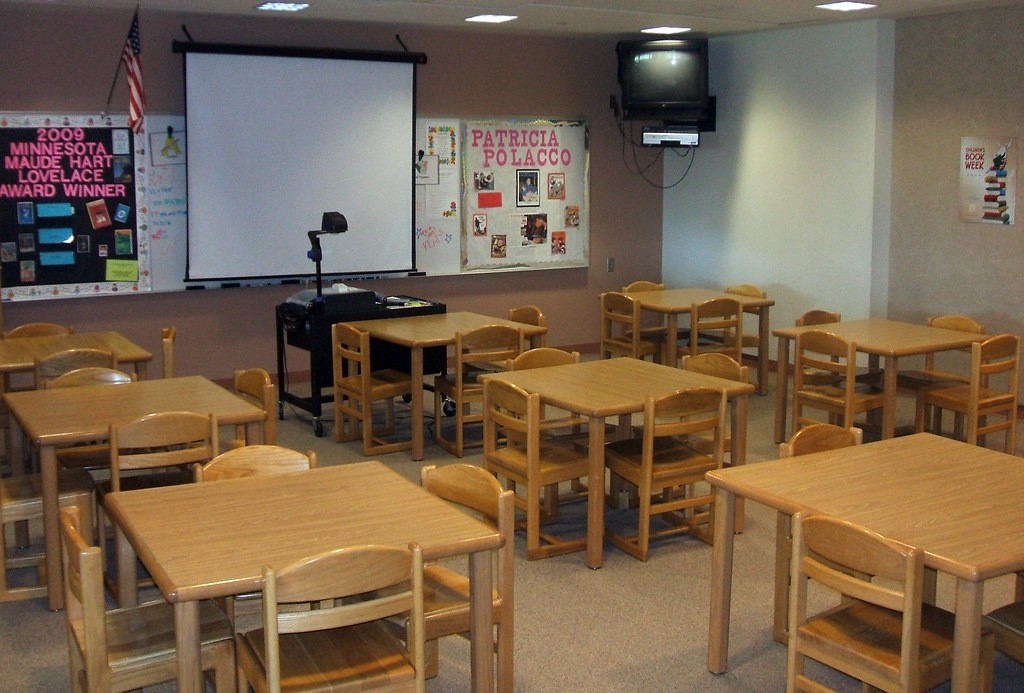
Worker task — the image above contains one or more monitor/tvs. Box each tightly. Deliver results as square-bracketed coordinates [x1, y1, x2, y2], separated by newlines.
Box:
[616, 38, 708, 111]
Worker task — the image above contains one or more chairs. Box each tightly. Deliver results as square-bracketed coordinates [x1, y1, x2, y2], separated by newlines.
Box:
[42, 368, 138, 492]
[604, 386, 727, 562]
[502, 347, 621, 524]
[983, 601, 1024, 665]
[192, 445, 321, 633]
[483, 378, 591, 562]
[22, 348, 117, 473]
[342, 464, 514, 693]
[1, 322, 77, 462]
[881, 316, 988, 444]
[621, 281, 691, 367]
[916, 332, 1021, 455]
[118, 327, 177, 474]
[794, 310, 885, 429]
[57, 506, 237, 693]
[433, 325, 525, 458]
[598, 291, 668, 366]
[698, 284, 769, 352]
[332, 323, 414, 457]
[785, 510, 994, 693]
[237, 542, 425, 693]
[629, 353, 749, 535]
[774, 423, 937, 645]
[187, 368, 276, 474]
[1, 465, 96, 602]
[791, 329, 885, 438]
[95, 412, 219, 609]
[467, 305, 547, 422]
[677, 298, 743, 365]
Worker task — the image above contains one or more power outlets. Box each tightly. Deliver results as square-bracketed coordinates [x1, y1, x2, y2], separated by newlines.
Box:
[607, 258, 616, 272]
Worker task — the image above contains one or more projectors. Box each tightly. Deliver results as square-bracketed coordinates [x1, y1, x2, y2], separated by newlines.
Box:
[286, 286, 376, 318]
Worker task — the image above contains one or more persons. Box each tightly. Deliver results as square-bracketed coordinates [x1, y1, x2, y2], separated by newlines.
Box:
[96, 212, 107, 223]
[472, 171, 581, 257]
[100, 246, 107, 256]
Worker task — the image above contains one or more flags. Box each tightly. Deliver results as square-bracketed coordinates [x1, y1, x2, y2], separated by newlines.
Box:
[120, 11, 146, 136]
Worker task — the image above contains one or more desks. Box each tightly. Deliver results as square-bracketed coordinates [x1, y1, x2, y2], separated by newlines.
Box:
[102, 460, 506, 693]
[476, 357, 756, 569]
[0, 329, 152, 473]
[622, 287, 775, 396]
[772, 318, 994, 444]
[0, 374, 269, 611]
[342, 311, 547, 462]
[703, 431, 1024, 693]
[275, 294, 448, 437]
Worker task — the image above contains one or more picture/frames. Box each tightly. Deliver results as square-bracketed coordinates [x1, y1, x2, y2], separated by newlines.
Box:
[548, 173, 565, 200]
[516, 169, 540, 208]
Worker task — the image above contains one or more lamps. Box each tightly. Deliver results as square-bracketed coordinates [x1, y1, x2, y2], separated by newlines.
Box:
[308, 210, 349, 295]
